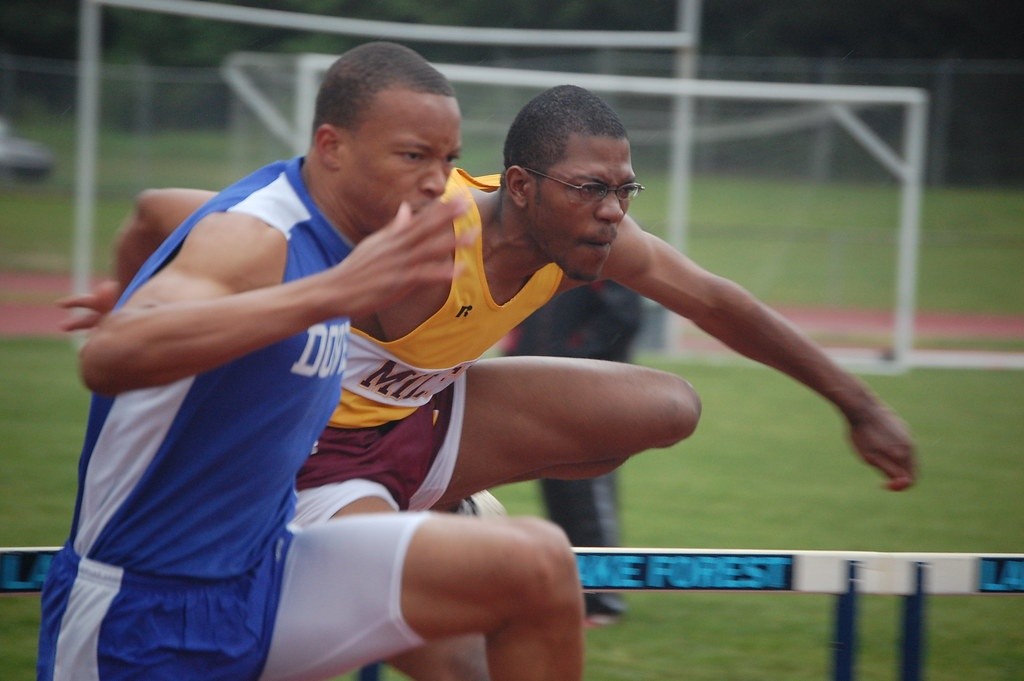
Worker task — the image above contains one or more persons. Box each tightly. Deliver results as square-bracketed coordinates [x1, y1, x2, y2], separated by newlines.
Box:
[35, 38, 589, 681]
[497, 241, 648, 629]
[53, 83, 920, 681]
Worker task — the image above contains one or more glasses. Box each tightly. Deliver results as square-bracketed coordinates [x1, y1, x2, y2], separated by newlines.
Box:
[515, 164, 644, 202]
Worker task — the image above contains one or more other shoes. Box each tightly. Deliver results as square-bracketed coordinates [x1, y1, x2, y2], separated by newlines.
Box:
[582, 614, 621, 629]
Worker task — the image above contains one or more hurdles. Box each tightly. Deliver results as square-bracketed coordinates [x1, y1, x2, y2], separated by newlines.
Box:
[0, 547, 1024, 681]
